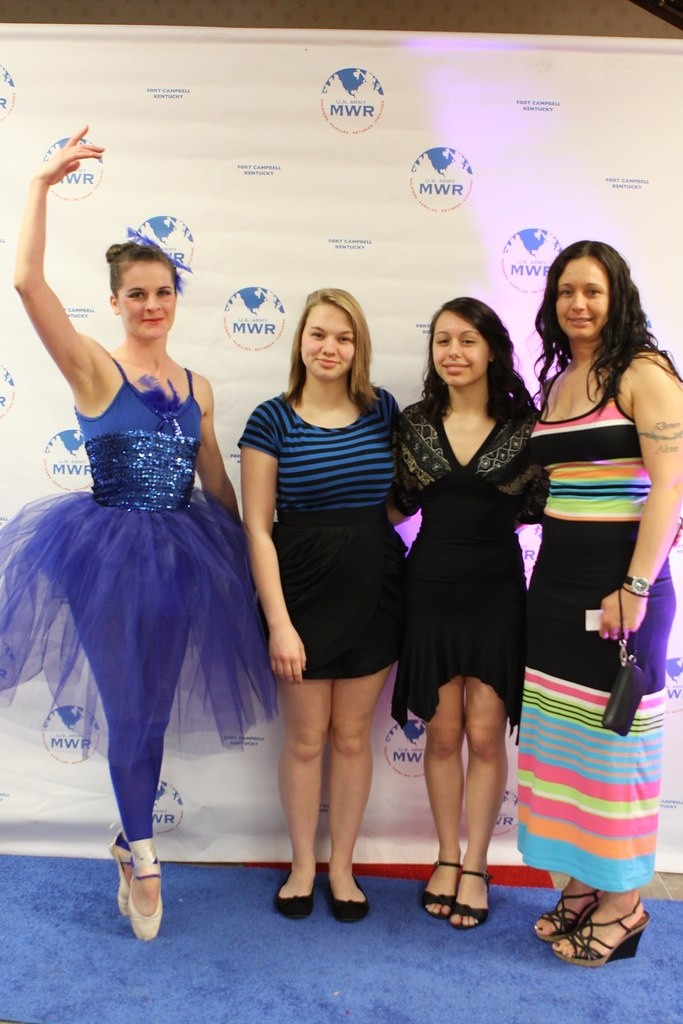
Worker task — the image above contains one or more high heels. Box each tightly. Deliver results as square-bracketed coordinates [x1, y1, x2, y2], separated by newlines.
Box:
[534, 889, 602, 944]
[551, 894, 650, 966]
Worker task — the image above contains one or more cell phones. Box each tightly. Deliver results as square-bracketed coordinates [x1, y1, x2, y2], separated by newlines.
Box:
[586, 610, 603, 631]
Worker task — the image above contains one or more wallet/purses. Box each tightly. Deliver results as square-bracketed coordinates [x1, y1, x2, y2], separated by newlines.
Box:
[602, 586, 648, 739]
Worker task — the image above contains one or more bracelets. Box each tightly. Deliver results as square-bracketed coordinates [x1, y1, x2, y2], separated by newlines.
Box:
[621, 585, 649, 598]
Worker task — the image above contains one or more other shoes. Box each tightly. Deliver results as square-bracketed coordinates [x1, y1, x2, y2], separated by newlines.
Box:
[107, 827, 131, 916]
[127, 853, 164, 941]
[328, 873, 369, 922]
[275, 871, 315, 918]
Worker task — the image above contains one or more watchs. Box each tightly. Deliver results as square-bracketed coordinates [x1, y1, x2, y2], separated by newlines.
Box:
[624, 576, 652, 593]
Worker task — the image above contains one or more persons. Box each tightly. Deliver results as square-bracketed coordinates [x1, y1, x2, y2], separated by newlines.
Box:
[516, 238, 681, 969]
[241, 288, 405, 925]
[386, 299, 548, 929]
[12, 123, 242, 942]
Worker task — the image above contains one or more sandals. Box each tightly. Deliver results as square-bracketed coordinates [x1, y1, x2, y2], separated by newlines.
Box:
[421, 860, 461, 920]
[449, 870, 490, 929]
